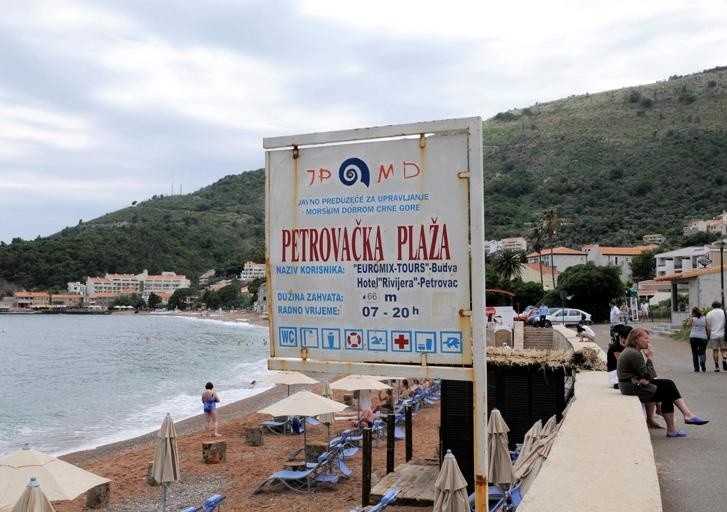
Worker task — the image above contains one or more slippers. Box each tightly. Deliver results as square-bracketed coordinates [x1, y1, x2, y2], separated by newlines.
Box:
[666, 430, 687, 437]
[648, 424, 665, 429]
[656, 410, 665, 417]
[685, 417, 709, 425]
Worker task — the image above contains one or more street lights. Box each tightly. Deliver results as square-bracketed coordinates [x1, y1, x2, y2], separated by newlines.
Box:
[712, 236, 727, 310]
[218, 307, 222, 320]
[543, 219, 556, 291]
[630, 276, 643, 289]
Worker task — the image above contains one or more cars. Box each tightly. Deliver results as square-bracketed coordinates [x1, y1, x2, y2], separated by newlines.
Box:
[533, 308, 593, 327]
[526, 307, 568, 326]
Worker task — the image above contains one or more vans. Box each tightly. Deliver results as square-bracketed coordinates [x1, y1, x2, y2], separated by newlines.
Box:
[513, 305, 538, 325]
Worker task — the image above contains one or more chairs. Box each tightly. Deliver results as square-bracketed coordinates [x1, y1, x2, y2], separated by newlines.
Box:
[464, 445, 524, 512]
[260, 415, 318, 436]
[254, 446, 347, 494]
[285, 414, 403, 484]
[390, 380, 441, 425]
[359, 487, 402, 512]
[173, 494, 227, 512]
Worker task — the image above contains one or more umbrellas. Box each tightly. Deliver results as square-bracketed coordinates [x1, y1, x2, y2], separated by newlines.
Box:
[329, 376, 394, 445]
[12, 475, 56, 512]
[268, 372, 320, 397]
[0, 442, 113, 512]
[256, 387, 350, 492]
[152, 413, 180, 512]
[432, 406, 559, 512]
[318, 380, 335, 474]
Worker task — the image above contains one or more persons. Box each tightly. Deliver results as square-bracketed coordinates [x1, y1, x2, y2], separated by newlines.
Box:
[354, 380, 430, 428]
[202, 382, 223, 437]
[538, 302, 549, 328]
[249, 380, 256, 389]
[576, 299, 727, 437]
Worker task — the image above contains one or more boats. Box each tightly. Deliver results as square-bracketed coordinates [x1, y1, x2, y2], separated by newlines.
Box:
[62, 304, 112, 315]
[0, 305, 60, 315]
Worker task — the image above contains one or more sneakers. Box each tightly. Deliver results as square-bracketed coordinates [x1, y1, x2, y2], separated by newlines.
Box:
[714, 368, 719, 372]
[722, 360, 727, 370]
